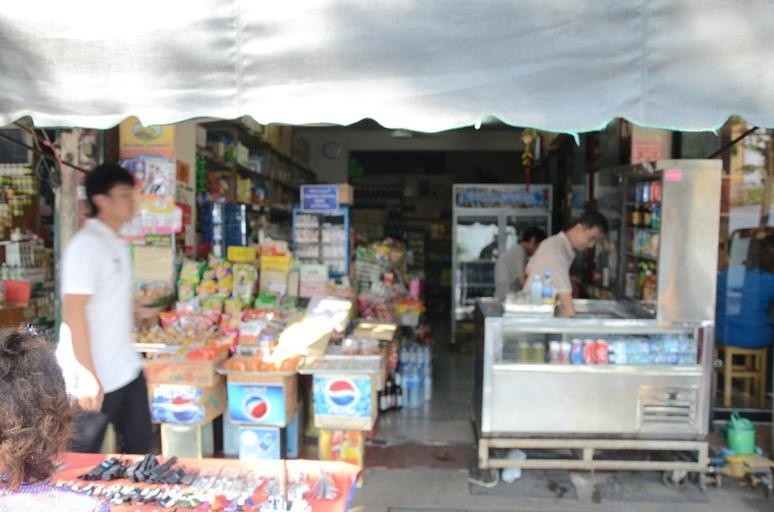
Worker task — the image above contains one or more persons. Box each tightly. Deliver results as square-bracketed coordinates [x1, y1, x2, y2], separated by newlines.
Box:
[524, 210, 609, 316]
[54, 164, 156, 454]
[493, 227, 548, 305]
[717, 241, 774, 398]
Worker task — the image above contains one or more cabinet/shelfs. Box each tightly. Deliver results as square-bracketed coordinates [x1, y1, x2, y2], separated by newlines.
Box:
[569, 159, 722, 322]
[720, 127, 774, 242]
[292, 206, 350, 277]
[192, 118, 317, 259]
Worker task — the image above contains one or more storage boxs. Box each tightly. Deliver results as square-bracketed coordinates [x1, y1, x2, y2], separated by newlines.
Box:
[142, 341, 389, 429]
[299, 182, 354, 209]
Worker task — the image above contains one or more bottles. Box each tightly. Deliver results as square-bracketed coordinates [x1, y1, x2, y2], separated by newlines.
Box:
[396, 345, 432, 408]
[609, 340, 695, 366]
[622, 262, 657, 304]
[630, 202, 661, 230]
[531, 276, 542, 304]
[502, 449, 527, 482]
[542, 271, 555, 305]
[377, 372, 401, 414]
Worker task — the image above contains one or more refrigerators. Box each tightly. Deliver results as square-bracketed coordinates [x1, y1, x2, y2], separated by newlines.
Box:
[449, 183, 552, 349]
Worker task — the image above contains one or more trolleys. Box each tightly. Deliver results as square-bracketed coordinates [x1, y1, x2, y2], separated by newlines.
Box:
[471, 297, 716, 491]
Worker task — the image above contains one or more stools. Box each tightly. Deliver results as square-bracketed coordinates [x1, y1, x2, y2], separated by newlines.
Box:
[713, 342, 769, 410]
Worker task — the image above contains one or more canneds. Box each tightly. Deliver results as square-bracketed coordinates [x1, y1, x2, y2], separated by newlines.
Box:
[517, 339, 608, 364]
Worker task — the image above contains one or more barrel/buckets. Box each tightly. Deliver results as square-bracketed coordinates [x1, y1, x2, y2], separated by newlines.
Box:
[725, 411, 757, 456]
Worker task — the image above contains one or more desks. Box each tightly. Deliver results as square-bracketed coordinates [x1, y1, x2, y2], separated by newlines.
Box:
[51, 452, 367, 512]
